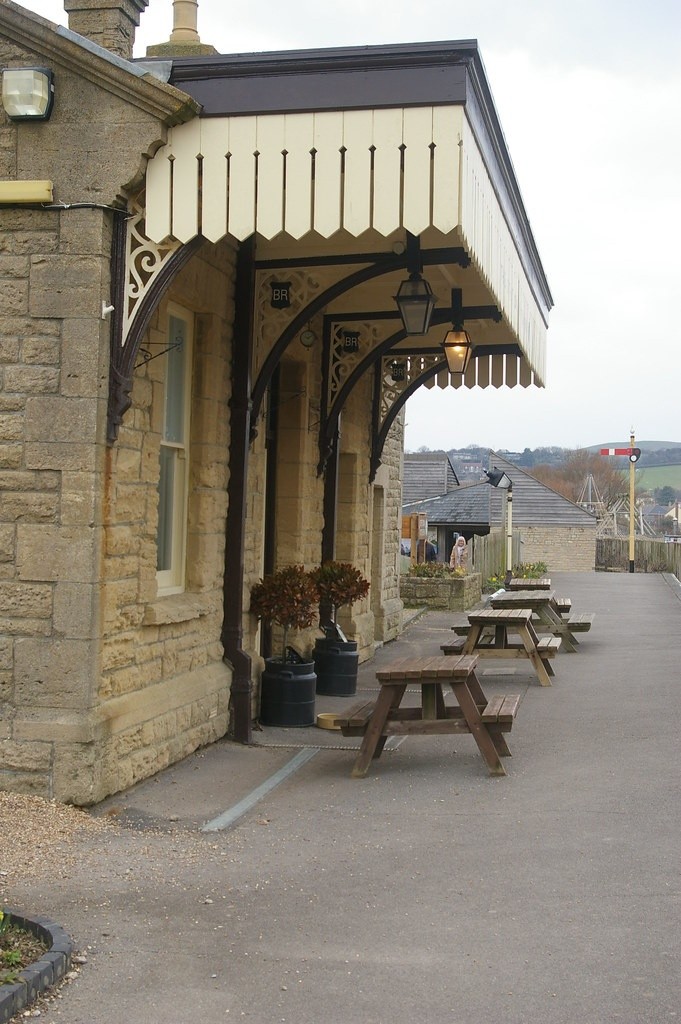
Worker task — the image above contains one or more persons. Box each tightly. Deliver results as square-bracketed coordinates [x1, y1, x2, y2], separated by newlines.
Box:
[450, 537, 467, 571]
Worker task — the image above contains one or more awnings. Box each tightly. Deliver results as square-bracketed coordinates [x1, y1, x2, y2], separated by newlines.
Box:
[119, 40, 556, 430]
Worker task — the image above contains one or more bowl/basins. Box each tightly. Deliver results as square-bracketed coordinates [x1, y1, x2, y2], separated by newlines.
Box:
[317, 713, 342, 729]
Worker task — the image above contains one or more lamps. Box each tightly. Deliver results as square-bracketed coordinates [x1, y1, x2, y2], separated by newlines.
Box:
[440, 318, 474, 376]
[392, 261, 438, 337]
[0, 67, 54, 122]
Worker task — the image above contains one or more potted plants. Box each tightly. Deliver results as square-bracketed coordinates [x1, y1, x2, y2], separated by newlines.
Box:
[250, 562, 323, 726]
[310, 560, 370, 697]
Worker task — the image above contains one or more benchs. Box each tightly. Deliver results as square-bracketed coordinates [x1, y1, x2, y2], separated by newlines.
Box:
[481, 693, 520, 724]
[334, 594, 593, 742]
[567, 609, 594, 633]
[451, 617, 471, 632]
[536, 637, 562, 651]
[334, 698, 377, 727]
[557, 598, 571, 610]
[440, 636, 468, 650]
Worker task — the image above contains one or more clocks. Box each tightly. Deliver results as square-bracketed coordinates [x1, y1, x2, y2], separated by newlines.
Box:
[299, 322, 318, 351]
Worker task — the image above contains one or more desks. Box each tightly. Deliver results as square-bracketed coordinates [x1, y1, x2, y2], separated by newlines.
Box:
[490, 590, 578, 655]
[351, 655, 511, 779]
[458, 610, 556, 687]
[509, 578, 551, 590]
